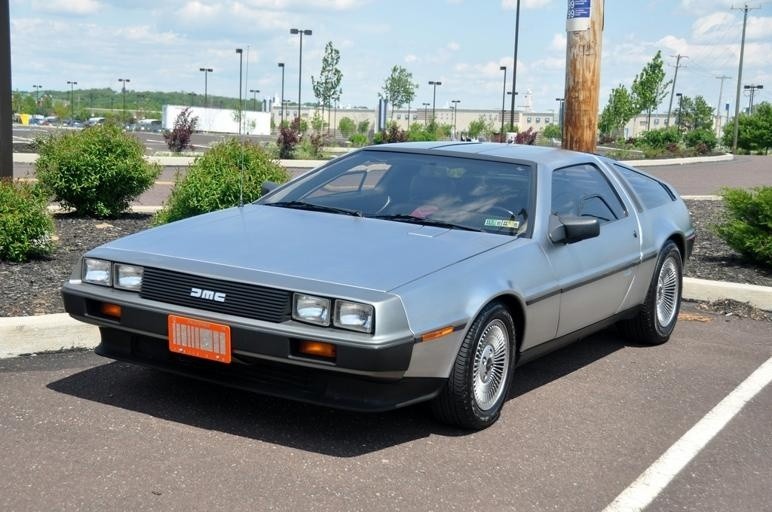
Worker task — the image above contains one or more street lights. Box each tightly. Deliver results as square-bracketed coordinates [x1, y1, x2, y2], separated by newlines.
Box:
[119, 78, 131, 124]
[32, 84, 44, 112]
[676, 93, 683, 134]
[289, 27, 314, 137]
[66, 80, 80, 119]
[744, 85, 765, 115]
[420, 64, 566, 149]
[187, 45, 287, 147]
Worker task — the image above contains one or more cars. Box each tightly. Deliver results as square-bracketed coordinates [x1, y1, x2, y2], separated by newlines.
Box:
[56, 136, 700, 436]
[15, 112, 163, 132]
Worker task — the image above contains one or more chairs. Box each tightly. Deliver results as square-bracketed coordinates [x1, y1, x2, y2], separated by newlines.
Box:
[387, 167, 456, 219]
